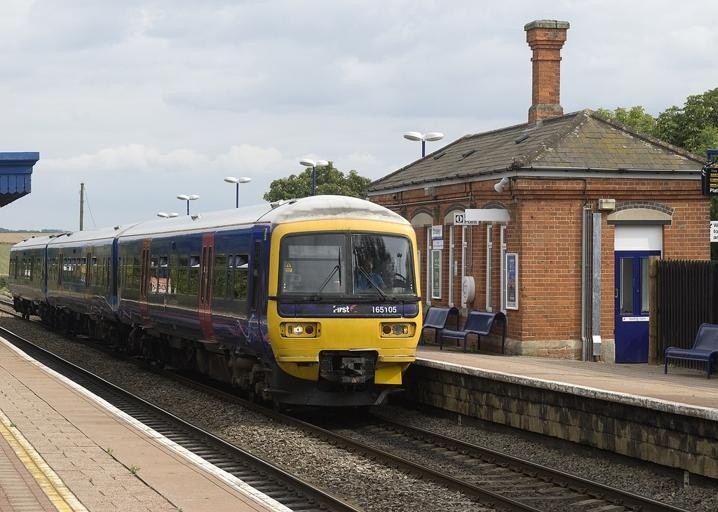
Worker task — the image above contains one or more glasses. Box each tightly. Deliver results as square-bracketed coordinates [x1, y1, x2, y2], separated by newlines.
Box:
[494, 178, 509, 194]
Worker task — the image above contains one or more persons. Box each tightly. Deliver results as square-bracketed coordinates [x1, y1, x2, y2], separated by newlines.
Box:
[354, 259, 387, 291]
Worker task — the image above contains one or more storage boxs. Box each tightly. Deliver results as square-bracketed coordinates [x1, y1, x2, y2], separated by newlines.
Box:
[664, 323, 718, 380]
[440, 311, 508, 355]
[421, 305, 459, 348]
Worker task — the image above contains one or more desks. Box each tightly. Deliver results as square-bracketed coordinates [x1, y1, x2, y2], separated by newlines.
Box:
[299, 159, 329, 196]
[223, 176, 252, 209]
[403, 131, 444, 158]
[175, 194, 200, 216]
